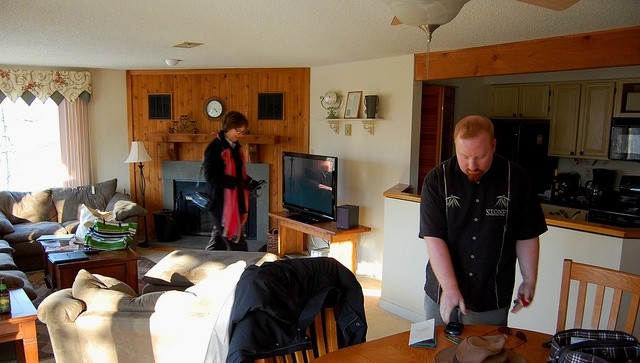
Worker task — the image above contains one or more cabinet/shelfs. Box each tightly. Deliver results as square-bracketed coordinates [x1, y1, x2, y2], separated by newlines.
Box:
[323, 118, 384, 135]
[548, 80, 616, 159]
[490, 84, 549, 120]
[144, 132, 279, 163]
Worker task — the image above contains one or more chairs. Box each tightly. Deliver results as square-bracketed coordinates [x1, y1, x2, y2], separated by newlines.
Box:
[555, 258, 639, 337]
[250, 256, 342, 363]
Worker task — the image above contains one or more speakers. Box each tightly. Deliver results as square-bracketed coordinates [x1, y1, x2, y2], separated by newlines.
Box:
[337, 204, 359, 229]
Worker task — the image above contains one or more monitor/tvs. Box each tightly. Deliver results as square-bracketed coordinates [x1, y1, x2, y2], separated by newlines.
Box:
[282, 151, 337, 224]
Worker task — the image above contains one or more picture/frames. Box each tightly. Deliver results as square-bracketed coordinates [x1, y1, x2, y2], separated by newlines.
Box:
[256, 91, 285, 121]
[146, 91, 173, 121]
[343, 91, 363, 119]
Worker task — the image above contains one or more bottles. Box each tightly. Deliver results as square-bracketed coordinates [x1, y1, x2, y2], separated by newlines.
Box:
[551, 168, 560, 196]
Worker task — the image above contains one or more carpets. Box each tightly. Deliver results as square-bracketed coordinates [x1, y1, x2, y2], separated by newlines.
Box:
[22, 256, 156, 360]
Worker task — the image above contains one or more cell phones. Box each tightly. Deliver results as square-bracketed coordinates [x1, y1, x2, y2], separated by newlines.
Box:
[444, 322, 463, 335]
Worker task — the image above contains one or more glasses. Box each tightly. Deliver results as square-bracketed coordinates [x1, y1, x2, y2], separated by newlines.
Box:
[235, 127, 246, 134]
[482, 326, 527, 349]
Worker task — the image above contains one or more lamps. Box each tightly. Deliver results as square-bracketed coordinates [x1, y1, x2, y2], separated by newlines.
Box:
[123, 140, 157, 249]
[382, 0, 470, 82]
[165, 60, 179, 67]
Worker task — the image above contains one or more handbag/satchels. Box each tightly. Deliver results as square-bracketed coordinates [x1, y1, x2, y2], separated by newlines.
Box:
[542, 329, 640, 363]
[73, 203, 116, 243]
[192, 162, 215, 210]
[84, 217, 139, 251]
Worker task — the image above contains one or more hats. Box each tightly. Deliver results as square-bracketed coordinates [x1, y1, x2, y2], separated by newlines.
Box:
[434, 334, 525, 362]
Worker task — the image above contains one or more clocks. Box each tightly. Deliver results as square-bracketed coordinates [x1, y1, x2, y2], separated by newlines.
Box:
[203, 96, 227, 122]
[323, 91, 338, 105]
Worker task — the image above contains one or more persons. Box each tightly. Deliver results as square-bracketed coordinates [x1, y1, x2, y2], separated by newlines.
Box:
[204, 111, 262, 252]
[419, 116, 540, 328]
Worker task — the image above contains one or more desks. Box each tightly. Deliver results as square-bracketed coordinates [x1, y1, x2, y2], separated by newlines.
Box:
[305, 322, 561, 363]
[1, 287, 40, 363]
[267, 210, 371, 275]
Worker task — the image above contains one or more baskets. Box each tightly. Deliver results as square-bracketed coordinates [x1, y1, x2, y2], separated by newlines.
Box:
[266, 228, 278, 253]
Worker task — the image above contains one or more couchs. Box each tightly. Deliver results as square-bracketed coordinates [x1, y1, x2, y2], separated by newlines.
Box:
[141, 248, 283, 296]
[0, 178, 148, 301]
[35, 268, 239, 363]
